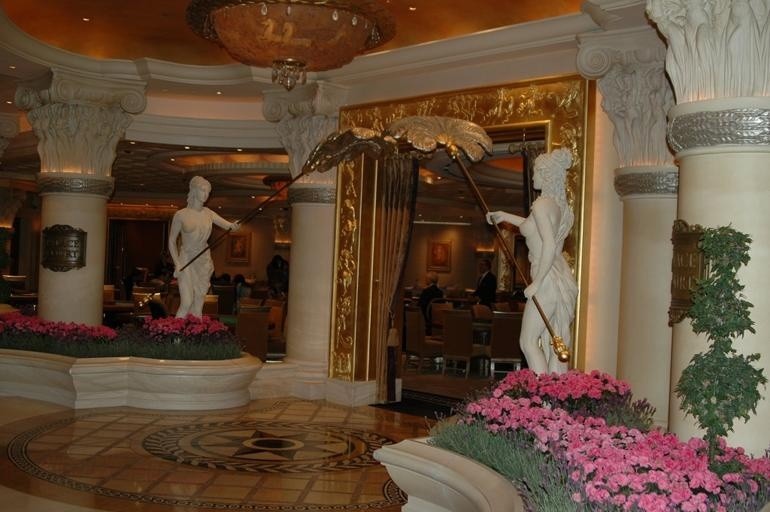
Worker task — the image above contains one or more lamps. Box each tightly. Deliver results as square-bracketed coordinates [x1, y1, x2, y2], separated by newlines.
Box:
[184, 0, 396, 93]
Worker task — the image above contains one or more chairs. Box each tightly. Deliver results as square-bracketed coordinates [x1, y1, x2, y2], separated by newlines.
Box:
[403, 285, 526, 382]
[104, 283, 288, 364]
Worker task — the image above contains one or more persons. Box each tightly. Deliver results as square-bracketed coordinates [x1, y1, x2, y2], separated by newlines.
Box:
[417, 271, 444, 336]
[486, 148, 578, 377]
[168, 176, 241, 318]
[471, 258, 497, 306]
[266, 255, 289, 298]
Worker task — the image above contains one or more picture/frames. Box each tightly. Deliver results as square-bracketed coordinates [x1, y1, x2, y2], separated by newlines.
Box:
[225, 231, 252, 267]
[426, 240, 452, 273]
